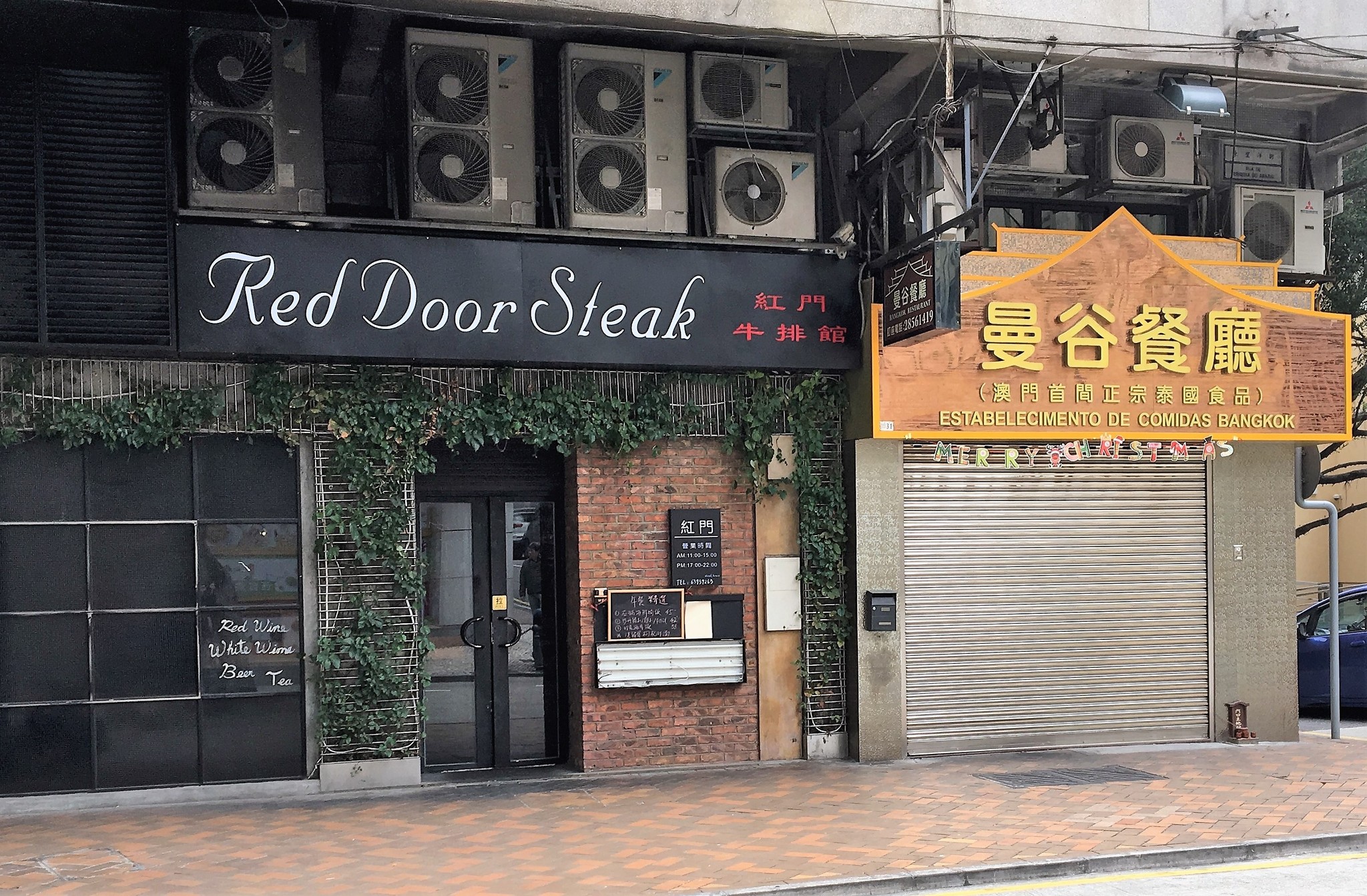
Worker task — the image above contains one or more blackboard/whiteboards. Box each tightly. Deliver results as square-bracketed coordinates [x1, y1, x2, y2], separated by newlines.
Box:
[607, 588, 684, 642]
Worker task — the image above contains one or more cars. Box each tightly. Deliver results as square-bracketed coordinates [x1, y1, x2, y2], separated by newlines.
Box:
[1297, 584, 1367, 719]
